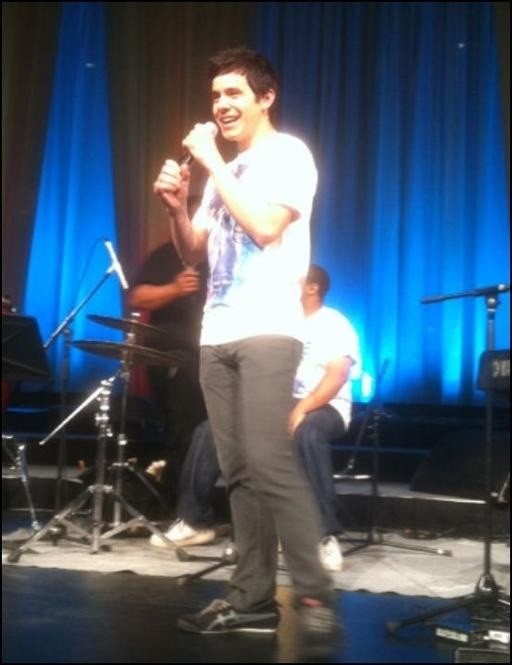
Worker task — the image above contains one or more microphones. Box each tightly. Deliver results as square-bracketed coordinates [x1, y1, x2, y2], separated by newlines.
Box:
[333, 475, 372, 480]
[178, 122, 218, 166]
[104, 241, 129, 289]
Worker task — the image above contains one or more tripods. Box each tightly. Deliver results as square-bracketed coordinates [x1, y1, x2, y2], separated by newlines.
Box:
[0, 433, 43, 544]
[4, 369, 194, 565]
[45, 311, 179, 552]
[338, 360, 451, 557]
[383, 284, 512, 635]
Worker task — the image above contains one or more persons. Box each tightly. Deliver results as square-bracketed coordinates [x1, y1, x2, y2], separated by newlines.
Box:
[150, 264, 362, 572]
[126, 194, 210, 457]
[153, 45, 344, 664]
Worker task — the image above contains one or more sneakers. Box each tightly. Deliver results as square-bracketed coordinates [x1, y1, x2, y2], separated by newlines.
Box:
[176, 597, 280, 636]
[291, 594, 344, 658]
[149, 518, 216, 549]
[317, 534, 344, 572]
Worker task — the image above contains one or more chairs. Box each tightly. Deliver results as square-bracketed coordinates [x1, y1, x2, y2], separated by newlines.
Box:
[0, 314, 93, 533]
[320, 357, 389, 561]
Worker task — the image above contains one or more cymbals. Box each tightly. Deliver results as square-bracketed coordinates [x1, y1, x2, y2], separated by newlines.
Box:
[86, 314, 171, 340]
[69, 340, 181, 366]
